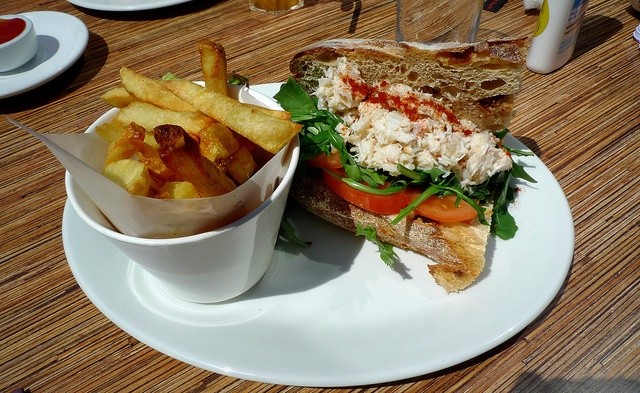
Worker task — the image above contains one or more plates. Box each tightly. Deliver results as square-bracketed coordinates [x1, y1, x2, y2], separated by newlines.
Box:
[60, 82, 576, 388]
[1, 10, 90, 101]
[64, 0, 196, 13]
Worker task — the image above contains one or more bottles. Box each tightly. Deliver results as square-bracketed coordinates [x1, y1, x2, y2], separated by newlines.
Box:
[526, 0, 590, 74]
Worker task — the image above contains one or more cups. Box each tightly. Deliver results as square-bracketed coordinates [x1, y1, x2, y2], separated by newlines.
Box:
[395, 0, 484, 46]
[0, 14, 39, 74]
[248, 0, 305, 16]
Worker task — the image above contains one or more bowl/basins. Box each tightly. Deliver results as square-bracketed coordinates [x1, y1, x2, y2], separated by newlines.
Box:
[64, 83, 300, 305]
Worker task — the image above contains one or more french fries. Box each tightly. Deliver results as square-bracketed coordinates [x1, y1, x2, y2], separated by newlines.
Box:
[94, 39, 303, 198]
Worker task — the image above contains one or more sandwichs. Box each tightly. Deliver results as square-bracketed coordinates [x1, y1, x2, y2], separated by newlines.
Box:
[272, 34, 538, 294]
